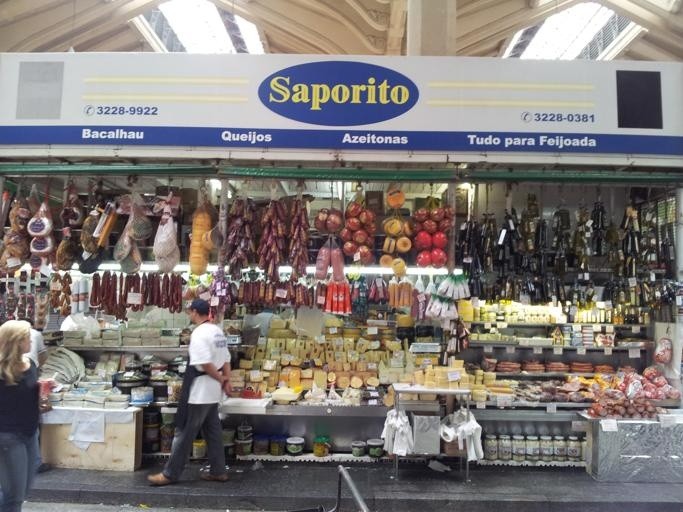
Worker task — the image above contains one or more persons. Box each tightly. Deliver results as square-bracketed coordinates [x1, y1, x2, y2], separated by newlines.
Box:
[0, 319, 40, 512]
[18, 319, 51, 473]
[146, 299, 234, 485]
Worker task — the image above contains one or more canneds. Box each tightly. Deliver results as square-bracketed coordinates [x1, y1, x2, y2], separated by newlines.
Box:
[484, 434, 588, 461]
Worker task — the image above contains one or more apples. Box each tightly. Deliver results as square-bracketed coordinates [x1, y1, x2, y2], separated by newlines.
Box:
[414, 204, 456, 268]
[315, 202, 377, 264]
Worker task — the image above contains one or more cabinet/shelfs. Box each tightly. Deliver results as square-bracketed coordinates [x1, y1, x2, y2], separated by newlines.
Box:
[217, 400, 441, 466]
[45, 345, 189, 461]
[451, 321, 657, 470]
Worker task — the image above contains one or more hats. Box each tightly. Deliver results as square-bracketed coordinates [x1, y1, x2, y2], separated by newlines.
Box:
[189, 299, 210, 313]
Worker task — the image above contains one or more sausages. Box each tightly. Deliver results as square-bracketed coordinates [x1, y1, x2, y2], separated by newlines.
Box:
[89, 270, 183, 322]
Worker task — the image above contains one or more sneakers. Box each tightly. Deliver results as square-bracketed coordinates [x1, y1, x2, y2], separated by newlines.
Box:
[201, 472, 228, 482]
[37, 462, 51, 473]
[147, 472, 171, 485]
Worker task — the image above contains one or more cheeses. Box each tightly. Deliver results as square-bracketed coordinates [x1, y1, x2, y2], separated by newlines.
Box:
[230, 318, 496, 403]
